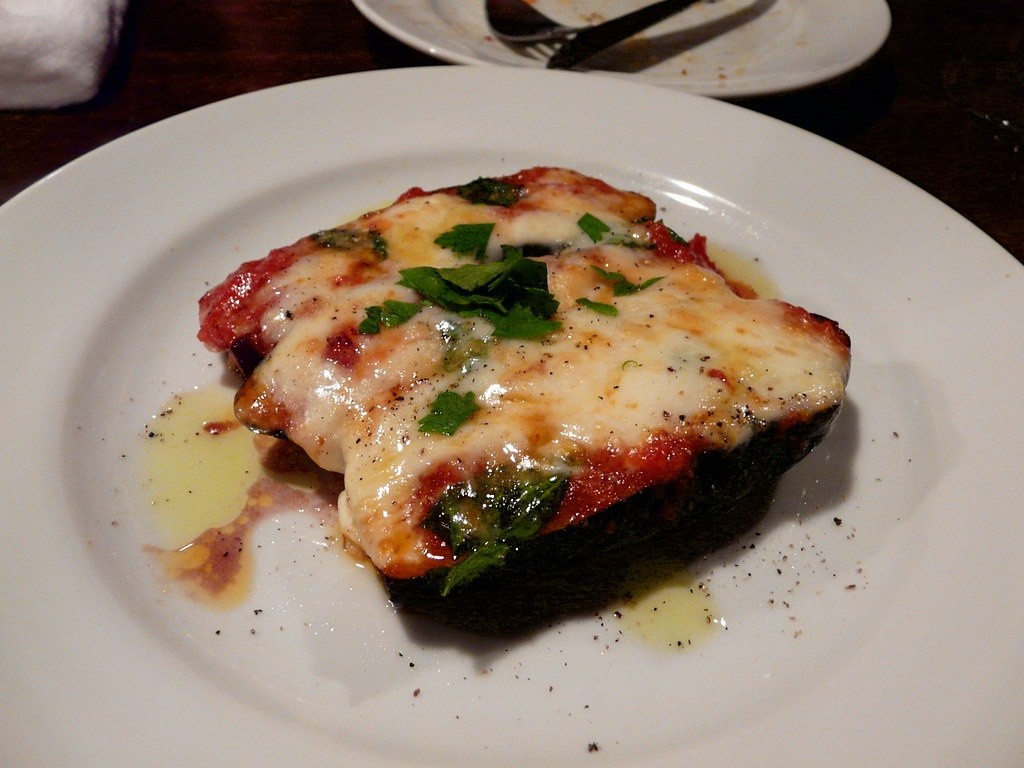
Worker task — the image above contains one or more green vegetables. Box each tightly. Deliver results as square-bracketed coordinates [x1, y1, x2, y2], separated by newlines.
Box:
[308, 173, 691, 600]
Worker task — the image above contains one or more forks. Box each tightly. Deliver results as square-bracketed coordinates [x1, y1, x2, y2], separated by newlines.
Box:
[483, 0, 594, 44]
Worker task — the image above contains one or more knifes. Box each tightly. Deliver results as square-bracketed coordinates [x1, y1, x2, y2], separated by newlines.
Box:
[547, 0, 712, 70]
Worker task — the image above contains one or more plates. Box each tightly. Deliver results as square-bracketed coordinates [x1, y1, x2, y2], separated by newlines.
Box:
[0, 60, 1024, 767]
[349, 0, 894, 98]
